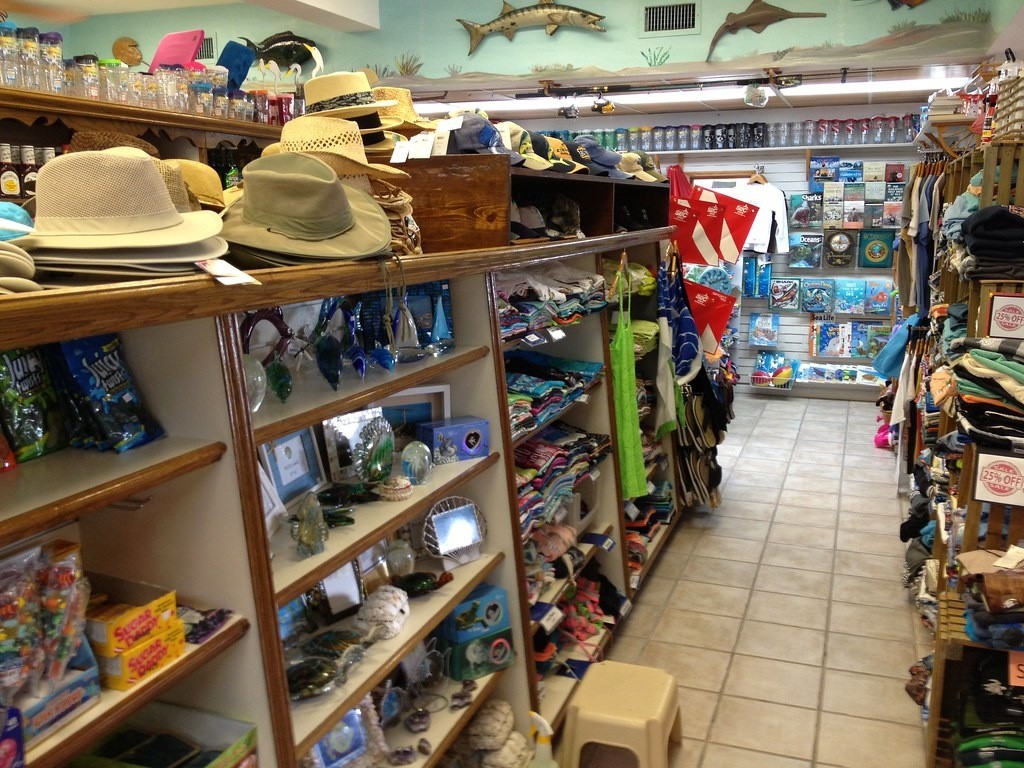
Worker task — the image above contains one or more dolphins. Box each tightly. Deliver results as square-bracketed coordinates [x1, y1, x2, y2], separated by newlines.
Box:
[313, 334, 344, 393]
[348, 345, 369, 379]
[367, 338, 398, 374]
[264, 350, 294, 404]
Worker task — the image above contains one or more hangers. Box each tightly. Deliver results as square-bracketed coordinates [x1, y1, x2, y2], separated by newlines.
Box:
[908, 148, 965, 179]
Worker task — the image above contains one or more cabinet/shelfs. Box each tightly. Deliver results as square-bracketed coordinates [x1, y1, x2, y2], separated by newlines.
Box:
[361, 149, 672, 256]
[0, 75, 285, 194]
[891, 56, 1023, 768]
[1, 225, 723, 768]
[392, 68, 972, 405]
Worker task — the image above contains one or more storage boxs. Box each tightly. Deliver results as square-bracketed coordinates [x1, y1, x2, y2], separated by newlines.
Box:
[9, 625, 103, 753]
[63, 566, 178, 660]
[93, 618, 185, 694]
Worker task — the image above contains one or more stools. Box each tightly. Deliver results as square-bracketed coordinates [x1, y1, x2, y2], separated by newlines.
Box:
[554, 655, 684, 768]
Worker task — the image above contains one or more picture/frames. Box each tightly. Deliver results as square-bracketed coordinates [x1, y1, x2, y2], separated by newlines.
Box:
[256, 459, 292, 544]
[422, 496, 486, 570]
[321, 406, 393, 484]
[258, 425, 328, 511]
[367, 385, 454, 457]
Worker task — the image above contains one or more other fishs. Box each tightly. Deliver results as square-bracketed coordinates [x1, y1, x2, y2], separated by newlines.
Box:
[772, 283, 799, 305]
[455, 0, 607, 57]
[238, 30, 316, 67]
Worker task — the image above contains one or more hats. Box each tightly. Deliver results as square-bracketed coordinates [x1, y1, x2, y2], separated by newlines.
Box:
[0, 73, 673, 297]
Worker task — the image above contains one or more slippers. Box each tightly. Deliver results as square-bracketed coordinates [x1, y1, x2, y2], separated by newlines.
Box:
[677, 376, 736, 510]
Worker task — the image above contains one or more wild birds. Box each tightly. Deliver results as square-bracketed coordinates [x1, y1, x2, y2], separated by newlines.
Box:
[284, 62, 304, 96]
[263, 60, 282, 90]
[257, 58, 267, 91]
[303, 43, 325, 78]
